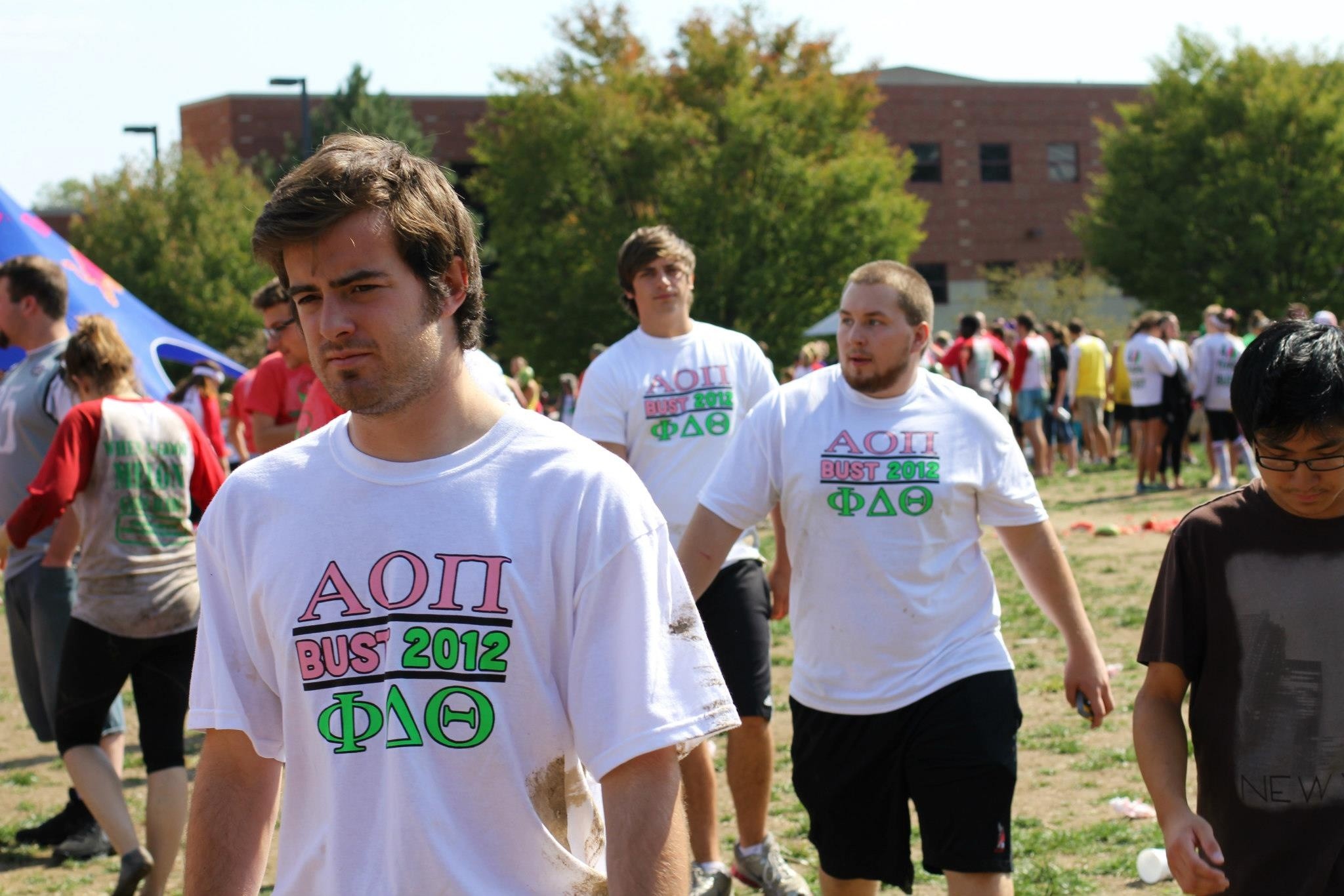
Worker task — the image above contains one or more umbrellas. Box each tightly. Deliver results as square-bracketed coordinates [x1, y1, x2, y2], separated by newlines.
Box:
[802, 310, 839, 337]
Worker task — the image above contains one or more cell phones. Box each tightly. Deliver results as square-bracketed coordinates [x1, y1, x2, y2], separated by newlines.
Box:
[1075, 688, 1094, 722]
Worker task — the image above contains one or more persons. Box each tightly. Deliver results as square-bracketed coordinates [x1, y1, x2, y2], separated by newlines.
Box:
[756, 339, 830, 384]
[463, 341, 609, 428]
[0, 256, 352, 896]
[927, 305, 1344, 493]
[181, 128, 741, 896]
[562, 223, 815, 896]
[677, 260, 1116, 896]
[1130, 318, 1344, 896]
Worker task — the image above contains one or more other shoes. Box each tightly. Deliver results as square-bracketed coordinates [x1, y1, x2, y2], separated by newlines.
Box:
[53, 822, 116, 858]
[15, 786, 95, 847]
[112, 846, 154, 896]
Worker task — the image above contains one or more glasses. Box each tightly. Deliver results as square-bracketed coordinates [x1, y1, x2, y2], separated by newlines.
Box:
[262, 318, 295, 339]
[1253, 438, 1344, 472]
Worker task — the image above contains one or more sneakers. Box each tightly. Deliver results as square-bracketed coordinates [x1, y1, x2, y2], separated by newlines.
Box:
[689, 861, 731, 896]
[732, 834, 812, 896]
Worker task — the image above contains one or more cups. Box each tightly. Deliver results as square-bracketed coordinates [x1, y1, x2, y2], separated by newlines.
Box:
[1135, 848, 1174, 885]
